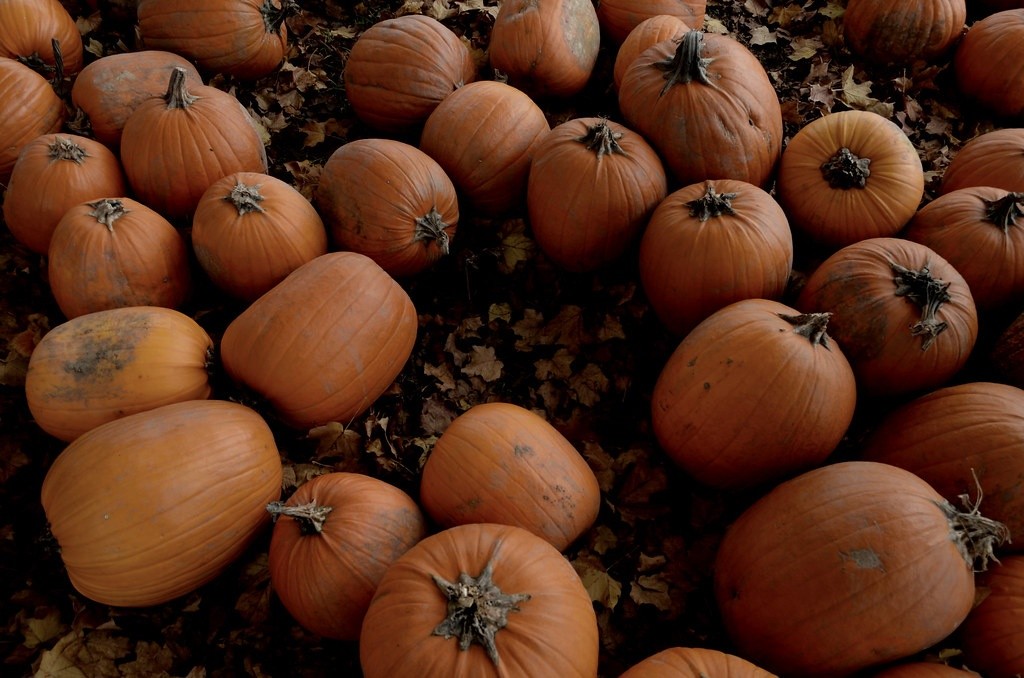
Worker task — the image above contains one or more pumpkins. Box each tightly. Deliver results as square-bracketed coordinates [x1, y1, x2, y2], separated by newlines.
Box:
[0, 0, 1024, 678]
